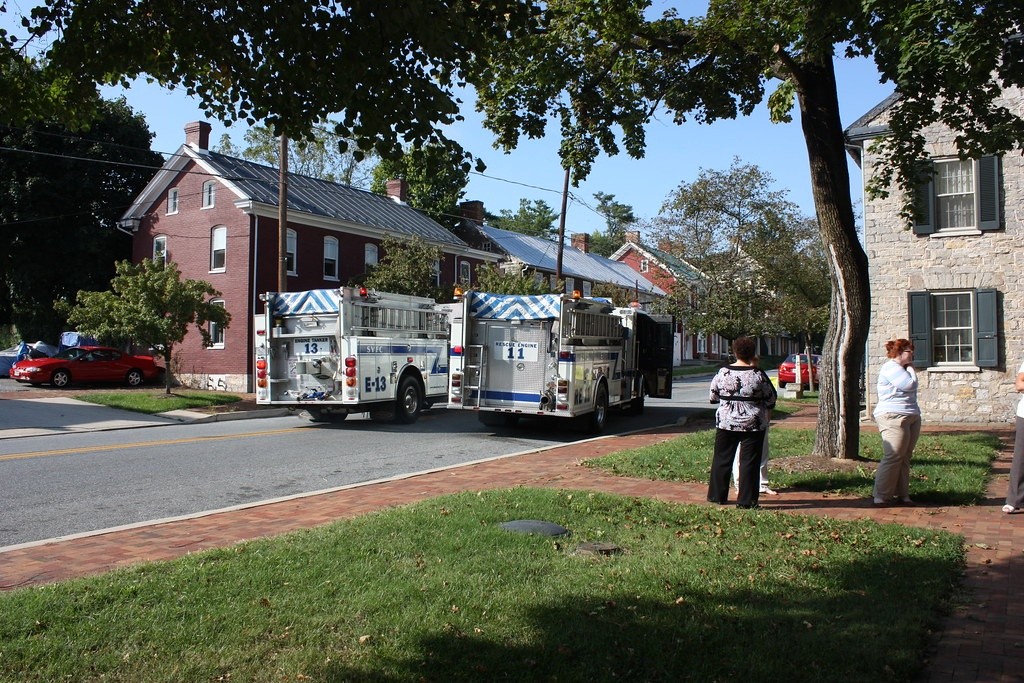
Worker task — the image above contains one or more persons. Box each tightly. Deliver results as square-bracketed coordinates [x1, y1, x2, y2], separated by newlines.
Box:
[1001, 361, 1024, 513]
[869, 339, 922, 508]
[706, 332, 777, 508]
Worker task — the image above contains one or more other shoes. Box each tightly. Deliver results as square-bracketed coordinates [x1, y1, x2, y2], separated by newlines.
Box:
[1002, 504, 1015, 513]
[707, 496, 726, 505]
[759, 484, 777, 495]
[872, 497, 891, 506]
[736, 503, 758, 509]
[896, 496, 916, 506]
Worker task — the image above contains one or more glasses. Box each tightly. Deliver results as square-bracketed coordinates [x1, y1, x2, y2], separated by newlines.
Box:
[904, 351, 914, 353]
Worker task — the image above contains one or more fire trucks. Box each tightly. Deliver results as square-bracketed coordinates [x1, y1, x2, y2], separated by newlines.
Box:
[249, 285, 464, 425]
[442, 284, 676, 436]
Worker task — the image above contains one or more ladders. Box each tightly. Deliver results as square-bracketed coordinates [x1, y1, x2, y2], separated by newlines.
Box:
[566, 308, 623, 341]
[350, 300, 451, 335]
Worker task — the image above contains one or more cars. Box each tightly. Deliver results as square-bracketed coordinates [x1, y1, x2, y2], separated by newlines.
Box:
[8, 345, 156, 387]
[778, 353, 821, 388]
